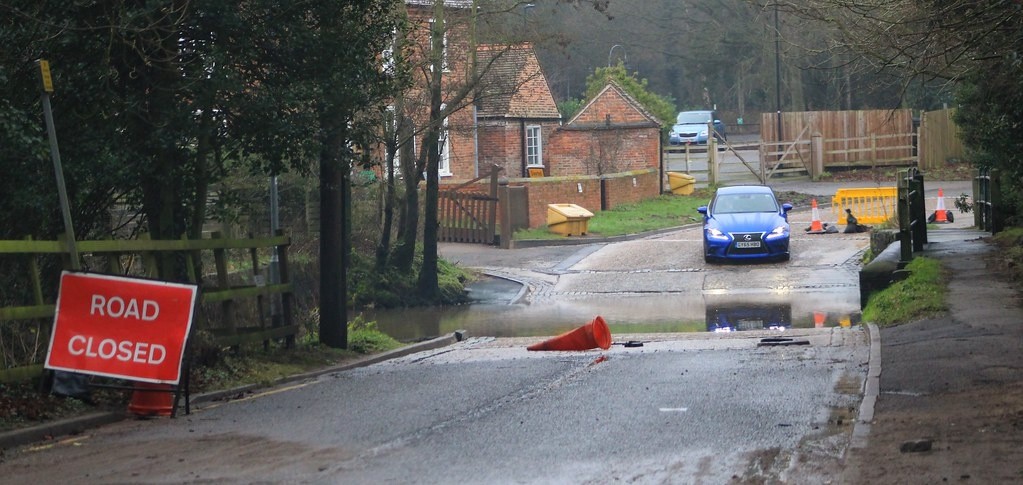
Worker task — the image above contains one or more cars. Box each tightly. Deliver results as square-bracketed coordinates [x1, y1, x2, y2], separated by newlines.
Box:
[697, 185, 794, 263]
[667, 111, 728, 150]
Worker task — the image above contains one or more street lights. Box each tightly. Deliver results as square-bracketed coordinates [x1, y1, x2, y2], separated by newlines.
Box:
[608, 45, 629, 76]
[523, 3, 537, 38]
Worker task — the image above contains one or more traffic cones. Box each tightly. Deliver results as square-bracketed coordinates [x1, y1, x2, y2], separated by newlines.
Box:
[128, 382, 175, 417]
[809, 200, 826, 234]
[528, 316, 611, 352]
[933, 188, 949, 223]
